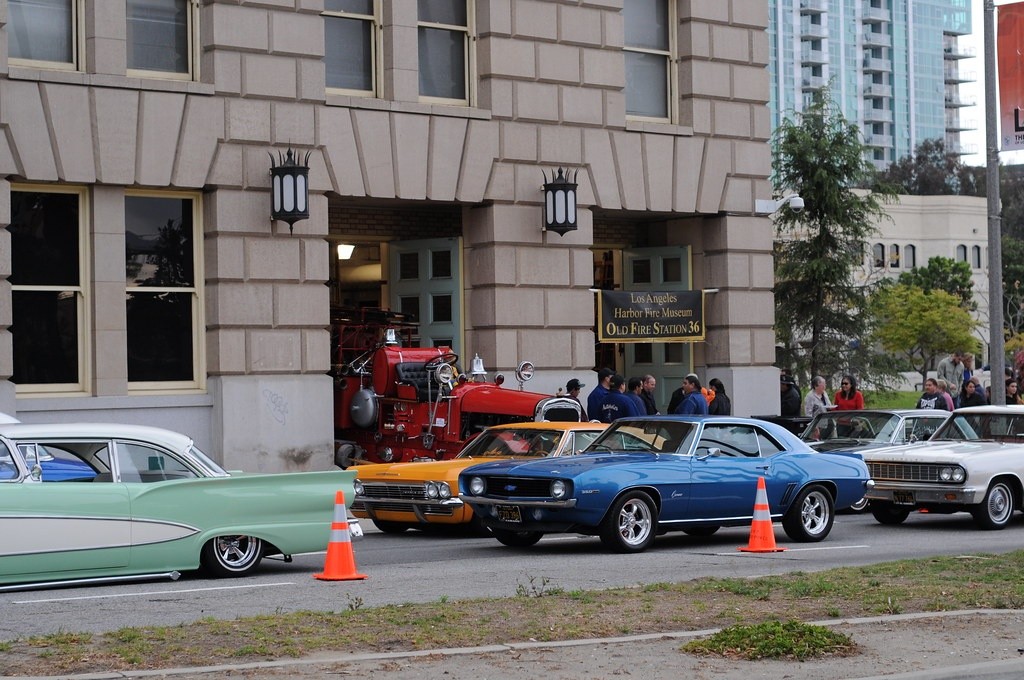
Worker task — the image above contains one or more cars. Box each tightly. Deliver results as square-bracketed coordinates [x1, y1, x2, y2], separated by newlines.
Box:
[344, 419, 669, 539]
[798, 408, 980, 514]
[456, 413, 875, 556]
[852, 404, 1024, 531]
[0, 413, 356, 593]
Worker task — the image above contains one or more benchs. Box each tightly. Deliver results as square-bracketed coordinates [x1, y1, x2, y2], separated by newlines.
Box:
[92, 470, 194, 483]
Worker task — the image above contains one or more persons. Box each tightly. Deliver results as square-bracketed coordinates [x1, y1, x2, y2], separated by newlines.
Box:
[566, 379, 588, 422]
[587, 368, 659, 422]
[938, 349, 973, 408]
[937, 380, 954, 412]
[913, 378, 947, 426]
[781, 369, 801, 418]
[803, 376, 837, 439]
[960, 364, 1024, 407]
[833, 375, 864, 435]
[667, 373, 731, 416]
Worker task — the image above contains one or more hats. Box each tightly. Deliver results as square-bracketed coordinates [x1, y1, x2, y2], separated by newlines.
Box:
[598, 367, 616, 378]
[565, 379, 585, 388]
[780, 375, 794, 385]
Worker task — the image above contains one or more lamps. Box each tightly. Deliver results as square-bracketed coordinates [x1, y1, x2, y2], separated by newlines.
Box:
[337, 244, 355, 260]
[539, 164, 580, 238]
[267, 138, 313, 239]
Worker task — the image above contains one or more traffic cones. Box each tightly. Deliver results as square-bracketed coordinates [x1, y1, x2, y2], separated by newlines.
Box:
[312, 488, 368, 580]
[734, 477, 789, 553]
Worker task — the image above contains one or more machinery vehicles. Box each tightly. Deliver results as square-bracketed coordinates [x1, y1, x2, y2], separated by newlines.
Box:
[329, 304, 581, 472]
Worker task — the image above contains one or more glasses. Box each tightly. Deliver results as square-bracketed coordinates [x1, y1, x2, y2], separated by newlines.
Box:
[842, 382, 850, 385]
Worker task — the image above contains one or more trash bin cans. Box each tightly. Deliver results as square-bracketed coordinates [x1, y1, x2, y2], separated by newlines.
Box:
[770, 415, 814, 437]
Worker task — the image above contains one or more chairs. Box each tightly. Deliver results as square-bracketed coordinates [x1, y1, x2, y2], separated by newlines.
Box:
[662, 438, 680, 453]
